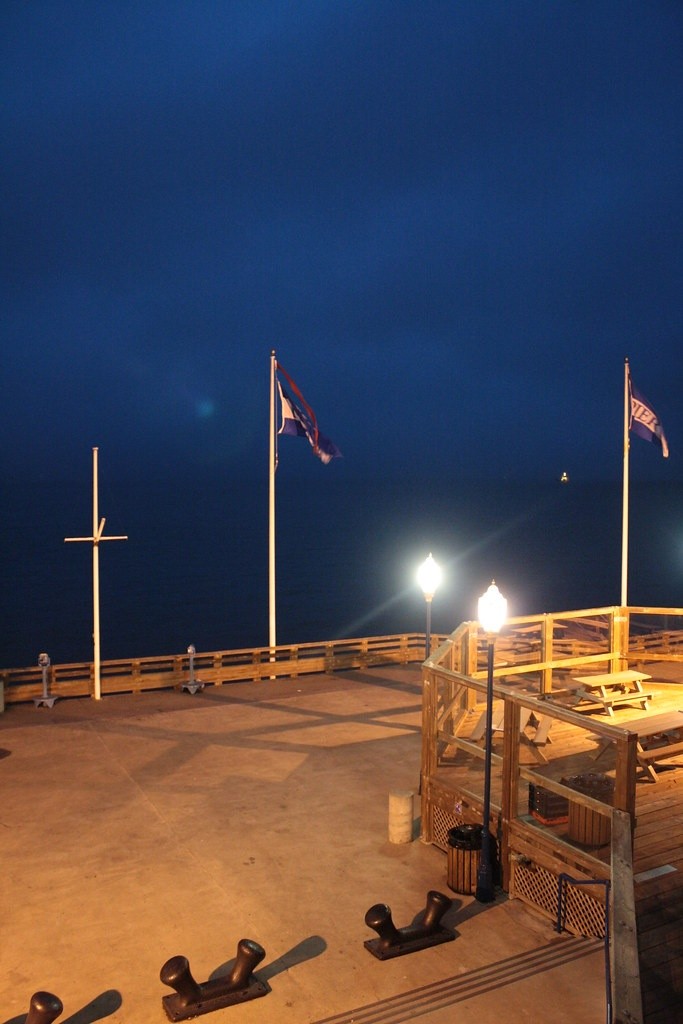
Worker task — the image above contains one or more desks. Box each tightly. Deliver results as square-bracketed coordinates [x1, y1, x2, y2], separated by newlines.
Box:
[588, 710, 683, 783]
[569, 670, 652, 716]
[499, 622, 568, 667]
[469, 697, 554, 767]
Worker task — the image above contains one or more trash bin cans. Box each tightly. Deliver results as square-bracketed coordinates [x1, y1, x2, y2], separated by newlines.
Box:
[447, 823, 490, 896]
[568, 773, 617, 847]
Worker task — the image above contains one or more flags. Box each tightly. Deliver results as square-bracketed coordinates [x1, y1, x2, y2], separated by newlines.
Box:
[276, 365, 342, 465]
[626, 378, 669, 459]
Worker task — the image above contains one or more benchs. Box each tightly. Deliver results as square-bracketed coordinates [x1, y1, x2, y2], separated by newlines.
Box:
[636, 742, 683, 763]
[533, 715, 553, 747]
[469, 710, 495, 743]
[597, 691, 661, 707]
[564, 683, 614, 695]
[586, 734, 605, 741]
[509, 636, 532, 643]
